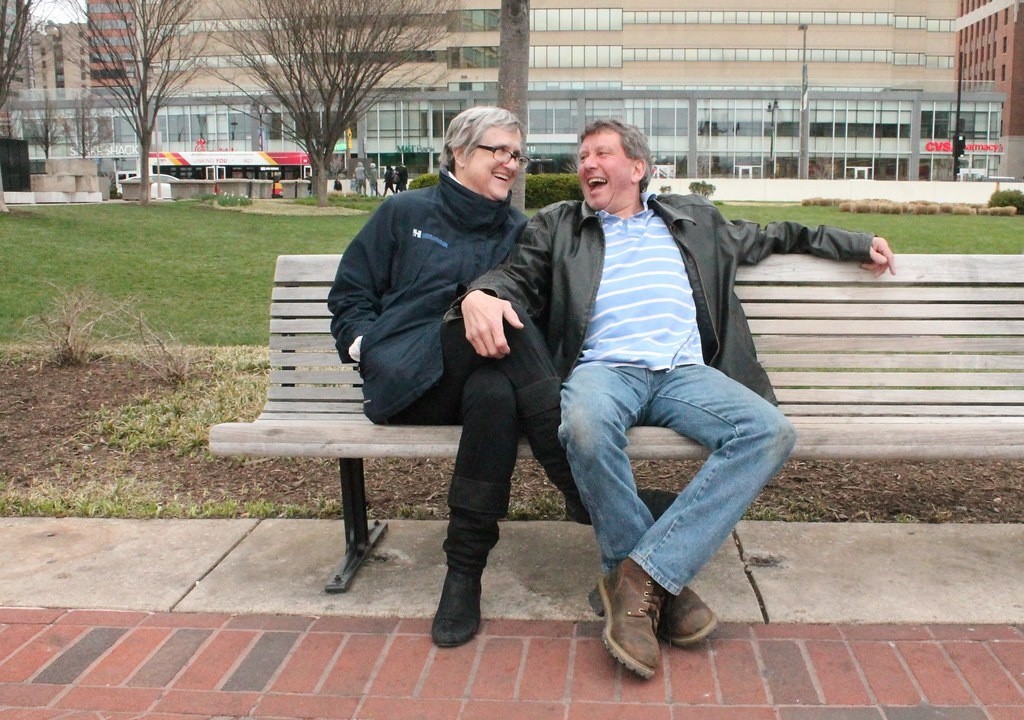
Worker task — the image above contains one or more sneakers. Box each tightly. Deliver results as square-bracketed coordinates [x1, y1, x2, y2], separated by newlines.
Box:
[658, 585, 717, 648]
[588, 557, 665, 680]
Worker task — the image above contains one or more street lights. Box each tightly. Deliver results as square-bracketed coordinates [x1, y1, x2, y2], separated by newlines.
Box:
[798, 24, 809, 179]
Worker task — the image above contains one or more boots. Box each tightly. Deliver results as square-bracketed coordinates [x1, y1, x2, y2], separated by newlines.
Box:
[525, 420, 679, 525]
[431, 511, 499, 646]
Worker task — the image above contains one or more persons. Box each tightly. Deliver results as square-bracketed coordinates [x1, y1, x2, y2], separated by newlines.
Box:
[327, 107, 679, 648]
[334, 162, 408, 197]
[442, 119, 896, 679]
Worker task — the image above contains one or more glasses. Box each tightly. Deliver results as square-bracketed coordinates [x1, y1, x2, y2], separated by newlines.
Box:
[478, 145, 530, 169]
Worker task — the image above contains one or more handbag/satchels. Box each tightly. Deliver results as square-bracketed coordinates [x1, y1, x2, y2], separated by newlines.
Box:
[351, 180, 357, 191]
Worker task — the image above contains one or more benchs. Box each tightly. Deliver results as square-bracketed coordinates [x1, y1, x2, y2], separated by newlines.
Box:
[208, 252, 1024, 594]
